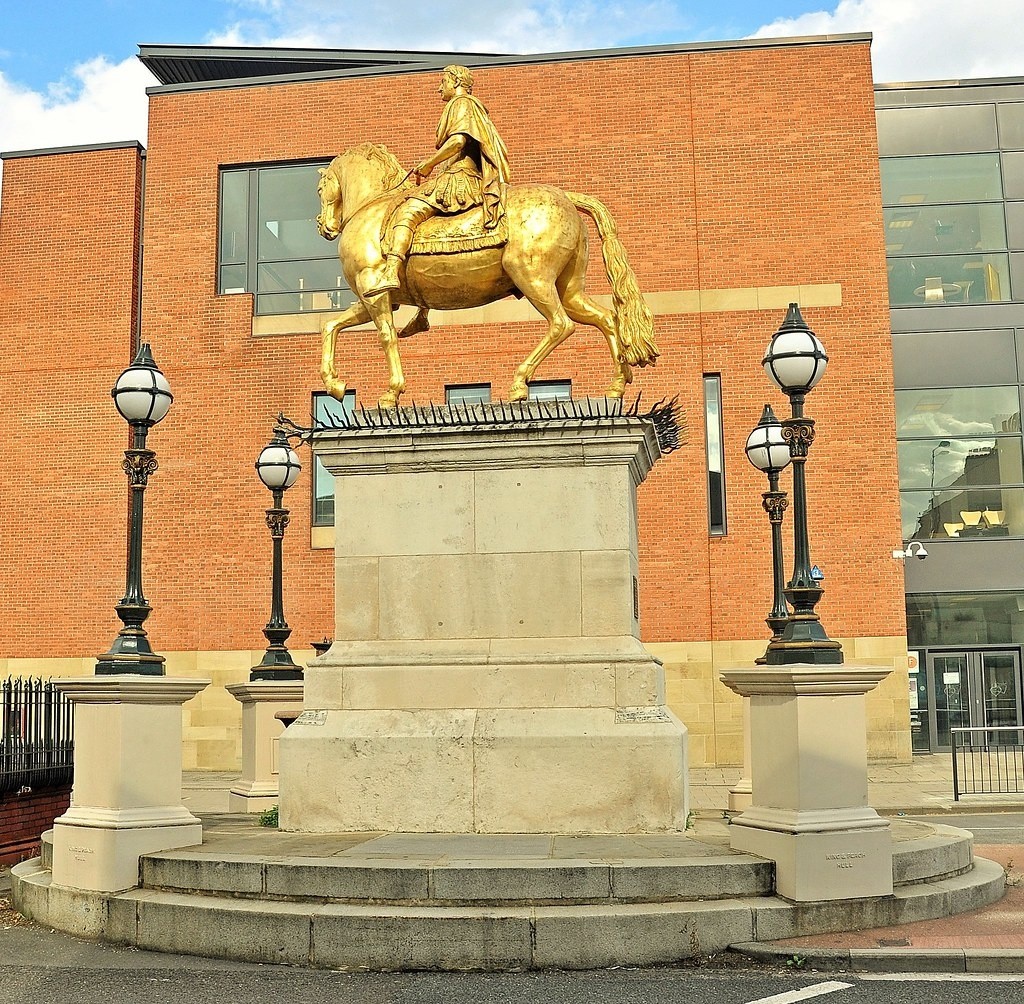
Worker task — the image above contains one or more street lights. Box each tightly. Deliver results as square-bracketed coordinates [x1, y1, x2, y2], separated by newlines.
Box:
[762, 301, 846, 665]
[95, 344, 173, 676]
[931, 439, 951, 538]
[251, 412, 305, 680]
[744, 404, 794, 664]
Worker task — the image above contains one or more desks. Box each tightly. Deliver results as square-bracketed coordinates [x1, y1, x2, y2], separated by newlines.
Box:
[913, 278, 961, 305]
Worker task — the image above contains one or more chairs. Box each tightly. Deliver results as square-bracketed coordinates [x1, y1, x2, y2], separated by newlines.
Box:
[942, 522, 964, 538]
[960, 510, 984, 538]
[925, 277, 945, 303]
[954, 281, 974, 303]
[981, 509, 1011, 536]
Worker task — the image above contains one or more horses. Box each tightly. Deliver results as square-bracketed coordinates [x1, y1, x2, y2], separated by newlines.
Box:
[315, 139, 662, 409]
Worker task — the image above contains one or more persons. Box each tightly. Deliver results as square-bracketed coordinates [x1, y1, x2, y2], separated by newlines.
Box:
[363, 65, 511, 338]
